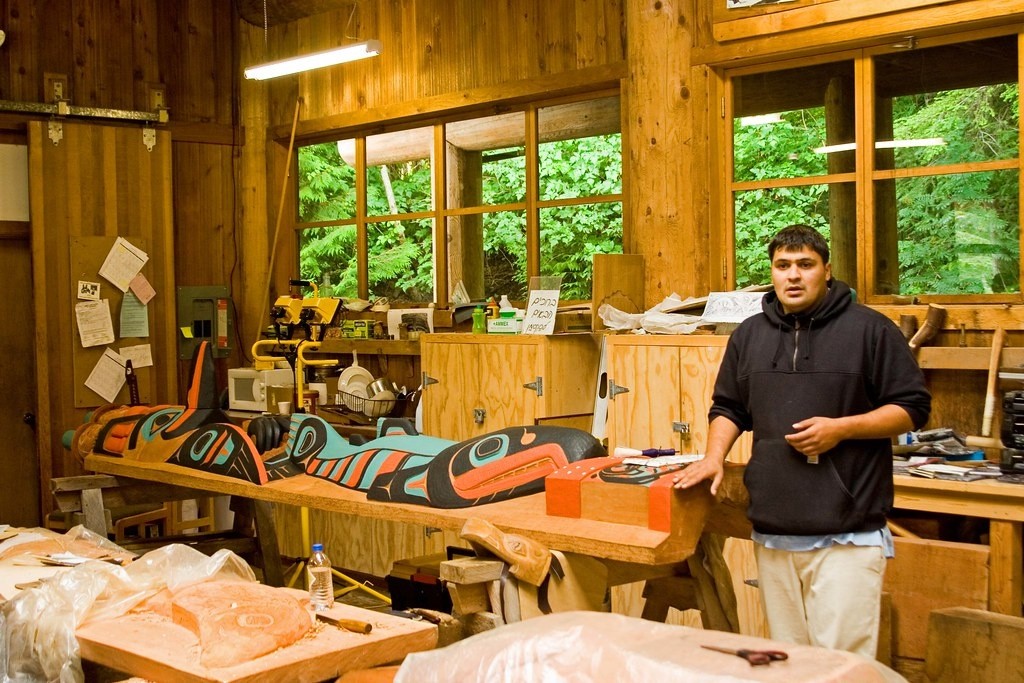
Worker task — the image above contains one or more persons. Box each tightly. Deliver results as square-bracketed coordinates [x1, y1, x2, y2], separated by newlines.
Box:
[671, 224, 932, 662]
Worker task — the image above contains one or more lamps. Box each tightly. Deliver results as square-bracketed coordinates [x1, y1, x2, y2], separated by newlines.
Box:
[244, 0, 379, 82]
[812, 135, 945, 158]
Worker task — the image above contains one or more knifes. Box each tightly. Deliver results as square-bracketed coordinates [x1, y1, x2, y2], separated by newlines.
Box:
[315, 613, 372, 634]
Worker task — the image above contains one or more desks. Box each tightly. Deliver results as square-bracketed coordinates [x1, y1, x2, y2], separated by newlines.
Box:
[1, 523, 441, 682]
[83, 413, 705, 563]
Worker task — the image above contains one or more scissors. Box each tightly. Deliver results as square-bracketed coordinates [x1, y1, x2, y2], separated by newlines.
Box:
[700, 642, 789, 666]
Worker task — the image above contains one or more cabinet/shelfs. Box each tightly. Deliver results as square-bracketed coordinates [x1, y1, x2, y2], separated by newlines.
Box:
[606, 334, 757, 492]
[229, 294, 427, 559]
[870, 304, 1022, 683]
[418, 329, 602, 442]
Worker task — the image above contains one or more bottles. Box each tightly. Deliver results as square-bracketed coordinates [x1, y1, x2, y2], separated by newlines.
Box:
[472, 305, 487, 333]
[308, 544, 334, 614]
[486, 296, 499, 319]
[499, 295, 512, 310]
